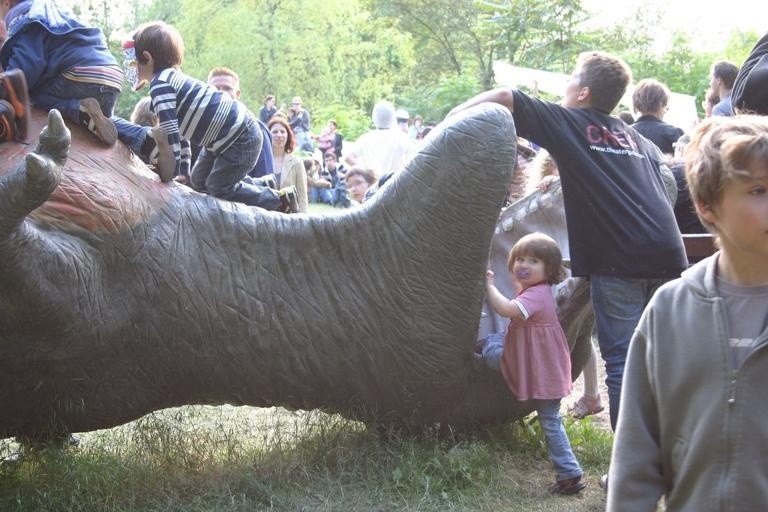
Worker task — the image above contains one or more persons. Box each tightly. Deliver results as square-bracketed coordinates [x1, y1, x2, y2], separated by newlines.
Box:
[524, 145, 604, 418]
[123, 20, 299, 213]
[620, 34, 768, 264]
[484, 231, 587, 498]
[444, 51, 690, 492]
[128, 65, 433, 207]
[605, 111, 768, 512]
[0, 0, 176, 182]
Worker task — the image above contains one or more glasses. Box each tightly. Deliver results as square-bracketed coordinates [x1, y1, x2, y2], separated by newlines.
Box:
[664, 105, 668, 113]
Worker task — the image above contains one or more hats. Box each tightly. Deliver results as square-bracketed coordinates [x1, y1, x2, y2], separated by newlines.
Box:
[371, 101, 394, 128]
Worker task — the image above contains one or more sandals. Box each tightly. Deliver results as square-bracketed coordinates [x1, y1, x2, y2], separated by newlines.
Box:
[549, 474, 586, 495]
[568, 394, 604, 419]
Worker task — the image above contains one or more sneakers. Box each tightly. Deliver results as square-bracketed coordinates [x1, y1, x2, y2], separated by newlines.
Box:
[0, 99, 17, 142]
[0, 68, 30, 139]
[262, 173, 278, 189]
[142, 128, 175, 183]
[280, 185, 300, 213]
[79, 97, 118, 145]
